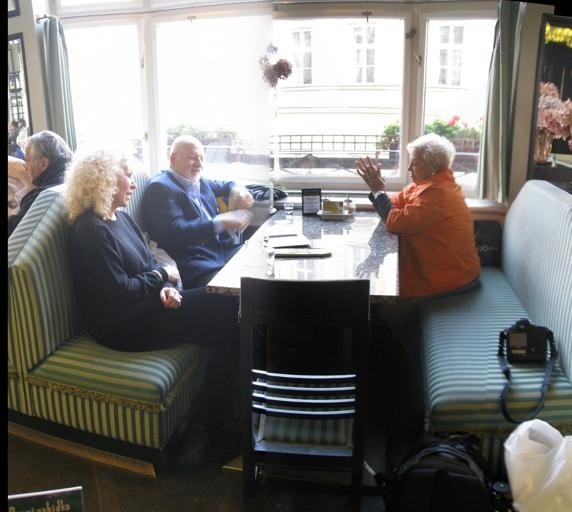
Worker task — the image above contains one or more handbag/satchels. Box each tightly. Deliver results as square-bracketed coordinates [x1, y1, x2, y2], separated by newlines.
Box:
[474, 219, 503, 268]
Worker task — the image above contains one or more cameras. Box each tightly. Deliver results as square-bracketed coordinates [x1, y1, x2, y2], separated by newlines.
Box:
[506, 317, 548, 363]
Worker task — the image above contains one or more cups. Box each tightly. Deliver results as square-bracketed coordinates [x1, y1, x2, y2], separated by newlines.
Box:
[264, 247, 276, 278]
[284, 203, 294, 216]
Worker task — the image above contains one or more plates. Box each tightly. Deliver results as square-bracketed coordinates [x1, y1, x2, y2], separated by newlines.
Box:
[316, 209, 352, 221]
[274, 248, 331, 257]
[264, 232, 312, 248]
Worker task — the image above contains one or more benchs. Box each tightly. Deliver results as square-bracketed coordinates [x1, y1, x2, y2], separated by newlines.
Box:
[6, 168, 207, 482]
[414, 179, 572, 481]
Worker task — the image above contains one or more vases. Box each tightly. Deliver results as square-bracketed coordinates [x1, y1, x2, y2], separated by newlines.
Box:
[534, 126, 554, 165]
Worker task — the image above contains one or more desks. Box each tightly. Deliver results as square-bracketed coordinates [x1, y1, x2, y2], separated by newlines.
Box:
[203, 208, 403, 493]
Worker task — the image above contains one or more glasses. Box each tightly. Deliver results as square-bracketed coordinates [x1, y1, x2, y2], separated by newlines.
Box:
[23, 154, 47, 169]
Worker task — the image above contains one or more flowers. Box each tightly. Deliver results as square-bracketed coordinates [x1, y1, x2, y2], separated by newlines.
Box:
[257, 41, 292, 88]
[533, 78, 571, 141]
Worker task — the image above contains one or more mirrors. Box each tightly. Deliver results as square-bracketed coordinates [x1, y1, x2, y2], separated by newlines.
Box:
[525, 12, 571, 181]
[7, 31, 32, 161]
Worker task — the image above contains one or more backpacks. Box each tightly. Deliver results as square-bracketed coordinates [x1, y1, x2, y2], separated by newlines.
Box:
[372, 439, 493, 512]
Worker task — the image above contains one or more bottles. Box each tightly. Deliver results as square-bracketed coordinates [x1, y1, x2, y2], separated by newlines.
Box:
[341, 199, 356, 216]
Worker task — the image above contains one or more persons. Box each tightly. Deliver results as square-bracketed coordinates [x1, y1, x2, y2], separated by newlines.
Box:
[141, 134, 255, 289]
[357, 131, 482, 346]
[65, 146, 242, 451]
[7, 128, 72, 237]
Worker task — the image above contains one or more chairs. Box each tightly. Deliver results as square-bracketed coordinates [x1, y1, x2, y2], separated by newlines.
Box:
[238, 278, 372, 511]
